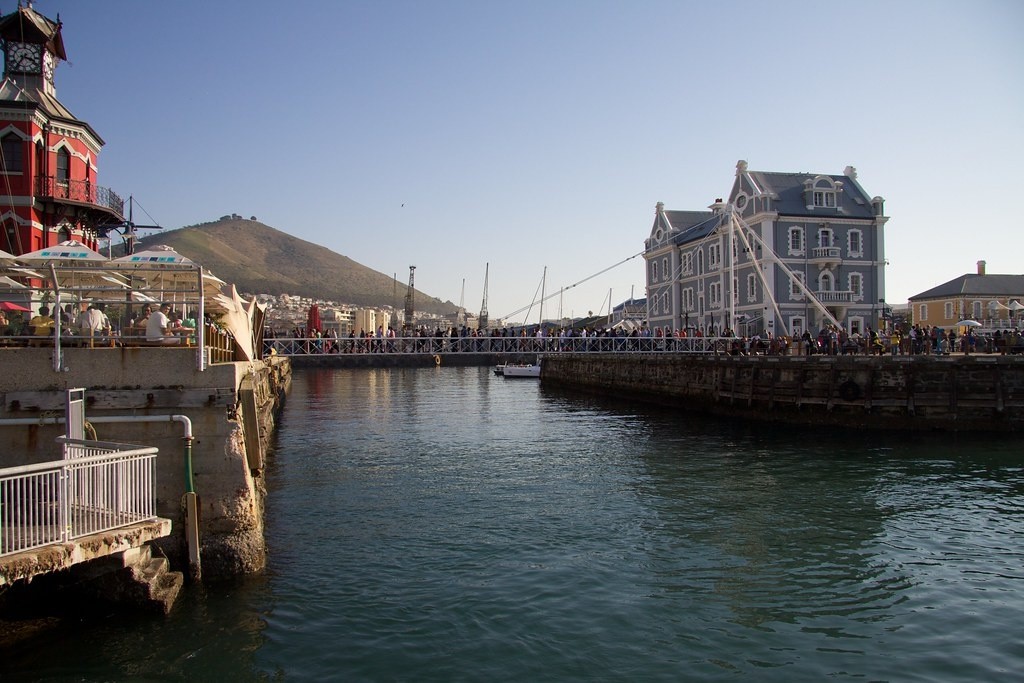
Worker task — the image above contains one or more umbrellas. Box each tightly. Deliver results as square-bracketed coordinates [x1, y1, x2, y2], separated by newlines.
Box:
[982, 300, 1024, 310]
[955, 320, 982, 326]
[0, 240, 228, 326]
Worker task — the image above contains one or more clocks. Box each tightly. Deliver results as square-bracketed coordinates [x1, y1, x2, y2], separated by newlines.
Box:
[5, 41, 43, 76]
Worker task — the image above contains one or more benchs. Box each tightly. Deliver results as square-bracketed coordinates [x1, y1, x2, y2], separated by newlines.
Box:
[120, 327, 235, 364]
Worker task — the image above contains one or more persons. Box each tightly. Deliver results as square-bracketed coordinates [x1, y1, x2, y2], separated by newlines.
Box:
[801, 324, 1024, 355]
[0, 303, 197, 346]
[291, 326, 787, 354]
